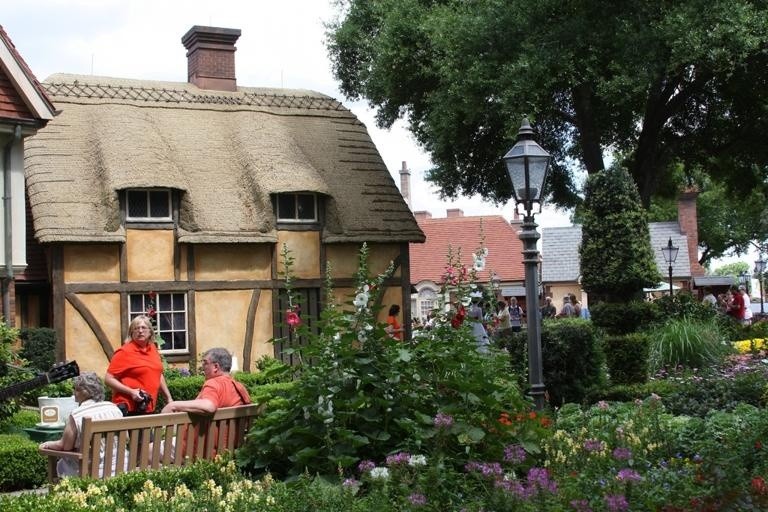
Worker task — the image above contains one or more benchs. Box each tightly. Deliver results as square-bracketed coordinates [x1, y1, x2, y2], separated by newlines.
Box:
[40, 405, 263, 492]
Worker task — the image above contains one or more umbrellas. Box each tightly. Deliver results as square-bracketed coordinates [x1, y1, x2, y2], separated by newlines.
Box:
[644, 281, 681, 296]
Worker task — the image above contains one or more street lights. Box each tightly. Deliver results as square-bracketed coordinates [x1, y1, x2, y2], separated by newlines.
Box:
[736, 269, 747, 285]
[503, 112, 555, 410]
[754, 252, 768, 313]
[660, 236, 679, 295]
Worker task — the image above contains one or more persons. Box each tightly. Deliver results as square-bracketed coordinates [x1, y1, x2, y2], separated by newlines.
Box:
[411, 294, 583, 355]
[104, 315, 173, 449]
[137, 347, 250, 468]
[388, 305, 405, 341]
[702, 284, 753, 327]
[647, 292, 656, 301]
[37, 371, 130, 479]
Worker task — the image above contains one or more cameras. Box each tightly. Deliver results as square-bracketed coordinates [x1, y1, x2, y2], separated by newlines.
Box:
[139, 392, 151, 411]
